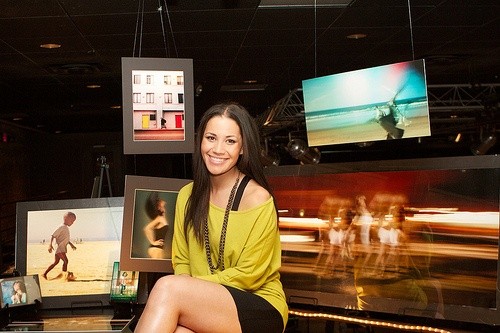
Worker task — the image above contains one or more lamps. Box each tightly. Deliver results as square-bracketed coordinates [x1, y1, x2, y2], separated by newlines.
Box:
[345, 12, 368, 41]
[36, 22, 75, 62]
[85, 71, 101, 88]
[287, 135, 322, 166]
[261, 136, 281, 168]
[471, 133, 496, 155]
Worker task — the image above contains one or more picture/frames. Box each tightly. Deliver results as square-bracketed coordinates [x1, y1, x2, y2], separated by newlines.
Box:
[122, 58, 196, 155]
[121, 176, 194, 274]
[15, 197, 148, 308]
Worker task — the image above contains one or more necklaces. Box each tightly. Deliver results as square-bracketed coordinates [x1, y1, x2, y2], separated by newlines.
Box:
[203, 171, 242, 274]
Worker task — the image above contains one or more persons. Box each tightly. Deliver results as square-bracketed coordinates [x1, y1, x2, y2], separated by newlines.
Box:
[144, 192, 170, 259]
[314, 192, 445, 319]
[144, 191, 170, 259]
[134, 102, 289, 333]
[41, 211, 76, 280]
[11, 280, 27, 304]
[120, 272, 128, 295]
[160, 117, 167, 130]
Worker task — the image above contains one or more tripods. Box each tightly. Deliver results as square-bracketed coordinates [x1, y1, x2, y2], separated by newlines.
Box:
[90, 155, 113, 198]
